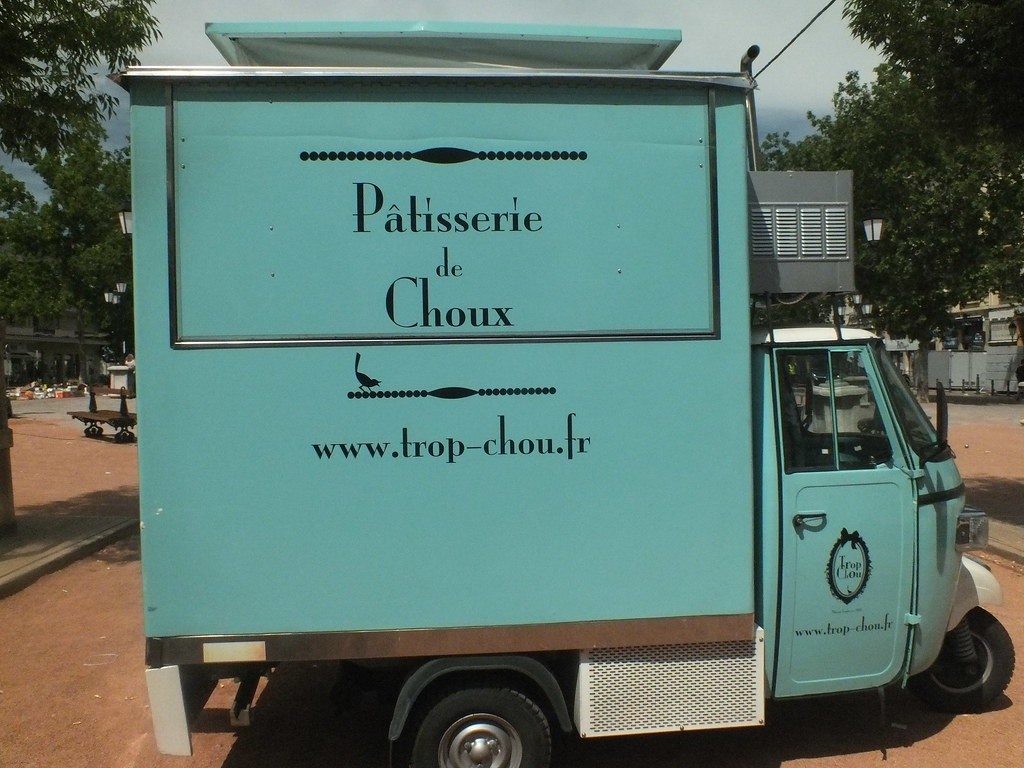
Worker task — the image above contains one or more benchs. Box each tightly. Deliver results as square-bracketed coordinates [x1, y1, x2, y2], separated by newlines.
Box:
[66, 385, 138, 442]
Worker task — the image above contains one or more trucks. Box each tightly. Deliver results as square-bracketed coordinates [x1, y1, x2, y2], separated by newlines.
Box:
[106, 17, 1017, 768]
